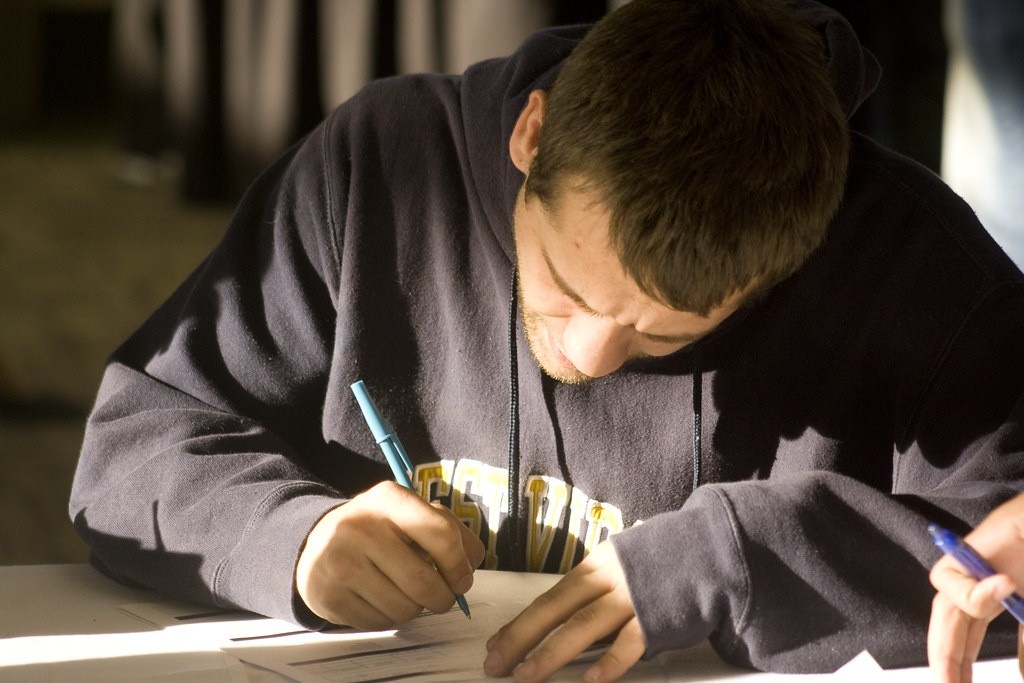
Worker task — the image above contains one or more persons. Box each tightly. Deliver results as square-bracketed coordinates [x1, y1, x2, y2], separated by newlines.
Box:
[69, 1, 1024, 683]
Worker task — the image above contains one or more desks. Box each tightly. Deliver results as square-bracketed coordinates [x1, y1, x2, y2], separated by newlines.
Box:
[0, 558, 937, 682]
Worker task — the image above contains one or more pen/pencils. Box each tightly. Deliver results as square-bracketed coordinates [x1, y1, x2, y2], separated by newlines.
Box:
[926, 523, 1024, 626]
[351, 380, 470, 622]
